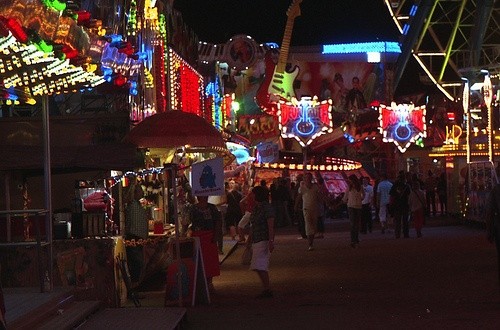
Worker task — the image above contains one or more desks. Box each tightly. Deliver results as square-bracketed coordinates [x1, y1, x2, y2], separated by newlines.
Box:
[143, 223, 176, 282]
[41, 235, 128, 308]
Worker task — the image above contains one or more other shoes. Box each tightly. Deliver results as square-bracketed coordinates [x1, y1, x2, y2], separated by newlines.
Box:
[237, 241, 245, 244]
[417, 233, 422, 238]
[297, 236, 305, 239]
[381, 227, 384, 233]
[351, 243, 355, 247]
[308, 245, 312, 250]
[218, 248, 223, 254]
[257, 290, 271, 297]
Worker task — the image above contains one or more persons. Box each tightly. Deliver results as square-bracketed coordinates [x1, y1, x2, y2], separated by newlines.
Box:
[180, 196, 224, 296]
[207, 174, 303, 265]
[298, 173, 330, 251]
[346, 76, 368, 109]
[251, 186, 276, 299]
[330, 173, 365, 248]
[124, 174, 150, 276]
[334, 73, 350, 110]
[318, 169, 447, 239]
[487, 164, 500, 284]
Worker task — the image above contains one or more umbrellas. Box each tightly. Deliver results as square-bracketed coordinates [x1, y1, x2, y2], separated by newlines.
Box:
[126, 110, 228, 152]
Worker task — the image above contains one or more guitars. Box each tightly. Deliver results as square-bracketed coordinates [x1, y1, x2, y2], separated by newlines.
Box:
[256, 0, 300, 117]
